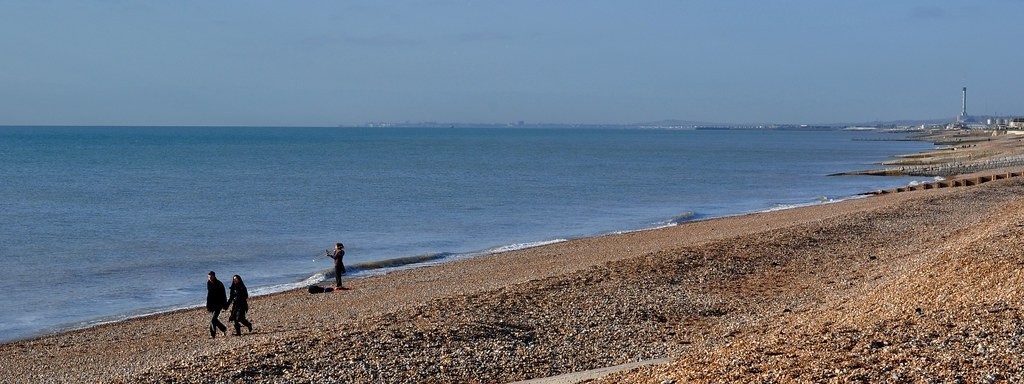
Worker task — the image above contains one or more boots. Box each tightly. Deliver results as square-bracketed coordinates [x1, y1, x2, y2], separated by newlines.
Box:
[242, 319, 252, 332]
[234, 324, 242, 336]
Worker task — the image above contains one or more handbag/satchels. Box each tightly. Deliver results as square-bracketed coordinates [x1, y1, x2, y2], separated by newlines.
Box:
[341, 263, 346, 274]
[309, 285, 324, 294]
[325, 287, 334, 292]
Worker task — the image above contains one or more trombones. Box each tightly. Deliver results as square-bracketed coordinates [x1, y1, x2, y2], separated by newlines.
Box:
[313, 249, 336, 262]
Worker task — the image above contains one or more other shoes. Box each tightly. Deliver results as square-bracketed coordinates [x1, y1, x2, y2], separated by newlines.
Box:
[222, 327, 228, 337]
[335, 286, 342, 290]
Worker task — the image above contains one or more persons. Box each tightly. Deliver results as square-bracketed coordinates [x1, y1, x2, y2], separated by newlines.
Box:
[326, 243, 346, 289]
[206, 271, 252, 339]
